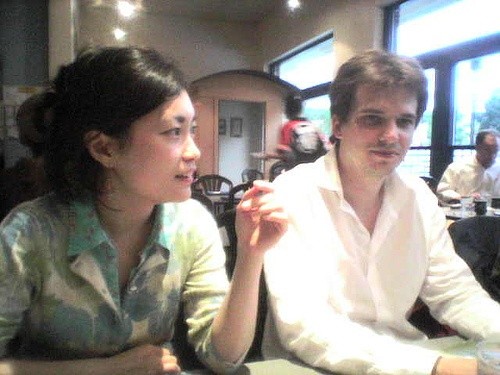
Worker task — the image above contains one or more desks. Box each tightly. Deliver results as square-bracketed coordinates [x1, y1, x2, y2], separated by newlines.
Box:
[175, 330, 473, 375]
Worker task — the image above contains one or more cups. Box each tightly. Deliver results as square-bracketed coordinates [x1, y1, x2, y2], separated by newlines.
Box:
[461, 196, 474, 211]
[491, 198, 500, 209]
[473, 200, 487, 216]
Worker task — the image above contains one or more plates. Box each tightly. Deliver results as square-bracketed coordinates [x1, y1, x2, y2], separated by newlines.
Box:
[493, 210, 500, 217]
[221, 196, 242, 200]
[446, 210, 477, 219]
[448, 203, 475, 208]
[207, 191, 223, 195]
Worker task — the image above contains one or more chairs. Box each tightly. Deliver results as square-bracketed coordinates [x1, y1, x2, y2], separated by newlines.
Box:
[188, 159, 289, 273]
[451, 216, 500, 302]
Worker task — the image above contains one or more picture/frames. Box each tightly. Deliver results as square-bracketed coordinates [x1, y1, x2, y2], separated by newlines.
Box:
[230, 117, 243, 137]
[218, 119, 226, 135]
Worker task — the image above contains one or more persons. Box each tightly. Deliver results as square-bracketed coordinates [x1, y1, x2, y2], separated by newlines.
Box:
[257, 49, 500, 375]
[436, 131, 500, 206]
[0, 43, 290, 375]
[247, 93, 331, 169]
